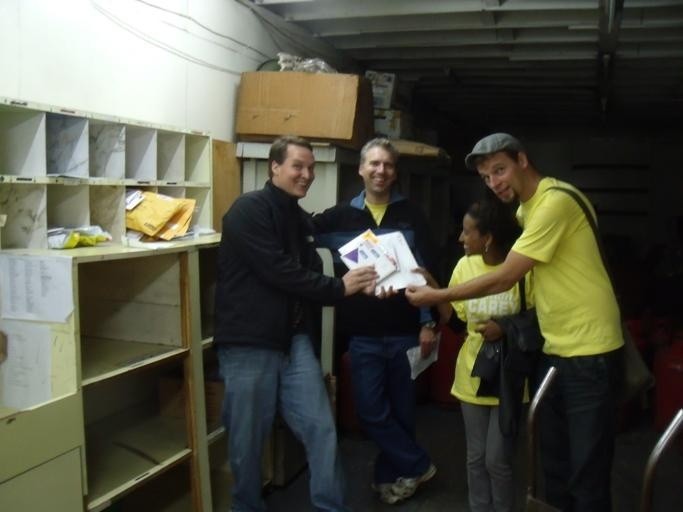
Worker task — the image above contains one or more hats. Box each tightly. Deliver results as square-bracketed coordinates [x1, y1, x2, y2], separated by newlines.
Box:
[466, 134, 523, 171]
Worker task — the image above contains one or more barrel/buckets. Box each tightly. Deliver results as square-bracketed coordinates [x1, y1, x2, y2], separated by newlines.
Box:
[339, 348, 371, 439]
[615, 314, 673, 431]
[652, 330, 683, 436]
[432, 311, 470, 411]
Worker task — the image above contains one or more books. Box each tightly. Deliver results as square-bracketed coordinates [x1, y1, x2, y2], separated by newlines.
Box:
[337, 229, 427, 298]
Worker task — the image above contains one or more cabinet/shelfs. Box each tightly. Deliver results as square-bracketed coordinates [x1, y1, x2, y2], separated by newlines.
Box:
[0, 95, 278, 512]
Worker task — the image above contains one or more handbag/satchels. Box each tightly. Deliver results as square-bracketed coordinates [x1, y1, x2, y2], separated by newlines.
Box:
[604, 323, 655, 435]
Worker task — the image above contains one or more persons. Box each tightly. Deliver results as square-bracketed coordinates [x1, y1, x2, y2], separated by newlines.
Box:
[403, 131, 627, 511]
[210, 136, 380, 512]
[447, 196, 531, 512]
[310, 137, 442, 505]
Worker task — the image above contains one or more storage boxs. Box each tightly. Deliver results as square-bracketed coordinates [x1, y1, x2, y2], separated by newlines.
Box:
[234, 66, 415, 152]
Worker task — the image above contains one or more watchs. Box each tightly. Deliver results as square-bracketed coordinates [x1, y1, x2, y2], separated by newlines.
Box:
[421, 319, 438, 330]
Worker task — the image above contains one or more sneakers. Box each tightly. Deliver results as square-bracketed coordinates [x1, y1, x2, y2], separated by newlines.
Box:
[370, 464, 437, 504]
